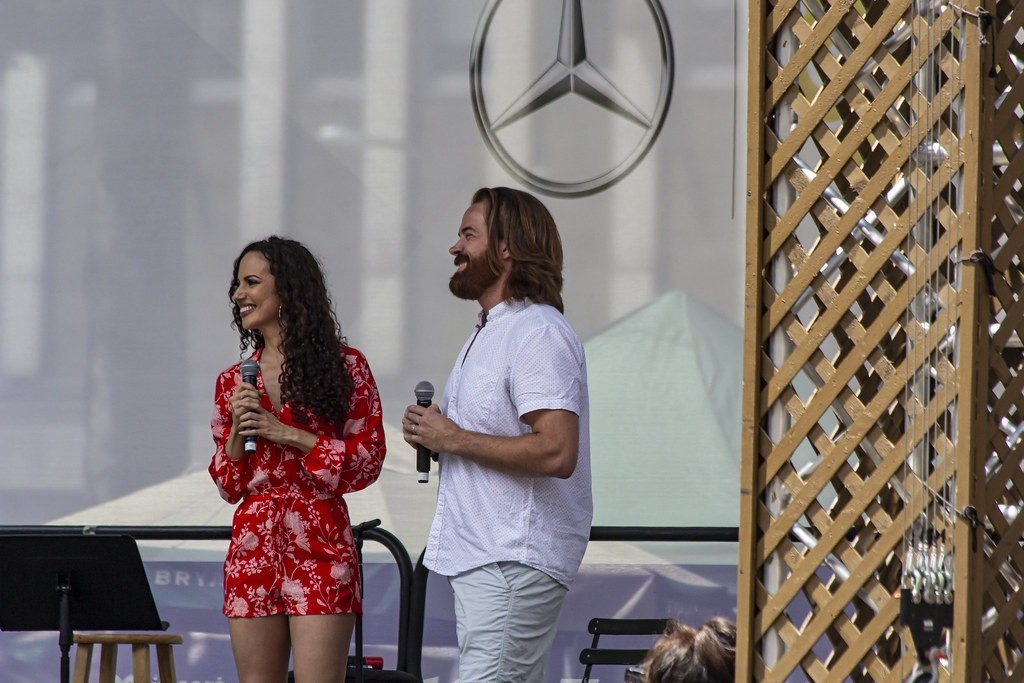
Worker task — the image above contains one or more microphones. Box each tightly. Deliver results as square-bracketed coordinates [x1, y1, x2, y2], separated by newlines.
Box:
[414, 381, 435, 484]
[241, 359, 260, 452]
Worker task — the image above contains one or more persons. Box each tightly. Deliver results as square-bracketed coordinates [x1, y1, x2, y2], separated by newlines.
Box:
[207, 233, 387, 683]
[639, 614, 737, 683]
[402, 187, 593, 683]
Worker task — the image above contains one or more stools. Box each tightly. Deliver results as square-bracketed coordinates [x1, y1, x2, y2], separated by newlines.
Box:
[73, 633, 183, 683]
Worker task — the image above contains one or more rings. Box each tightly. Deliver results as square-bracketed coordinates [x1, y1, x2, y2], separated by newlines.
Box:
[411, 425, 417, 435]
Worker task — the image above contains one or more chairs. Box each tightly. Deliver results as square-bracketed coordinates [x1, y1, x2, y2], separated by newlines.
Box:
[578, 618, 674, 683]
